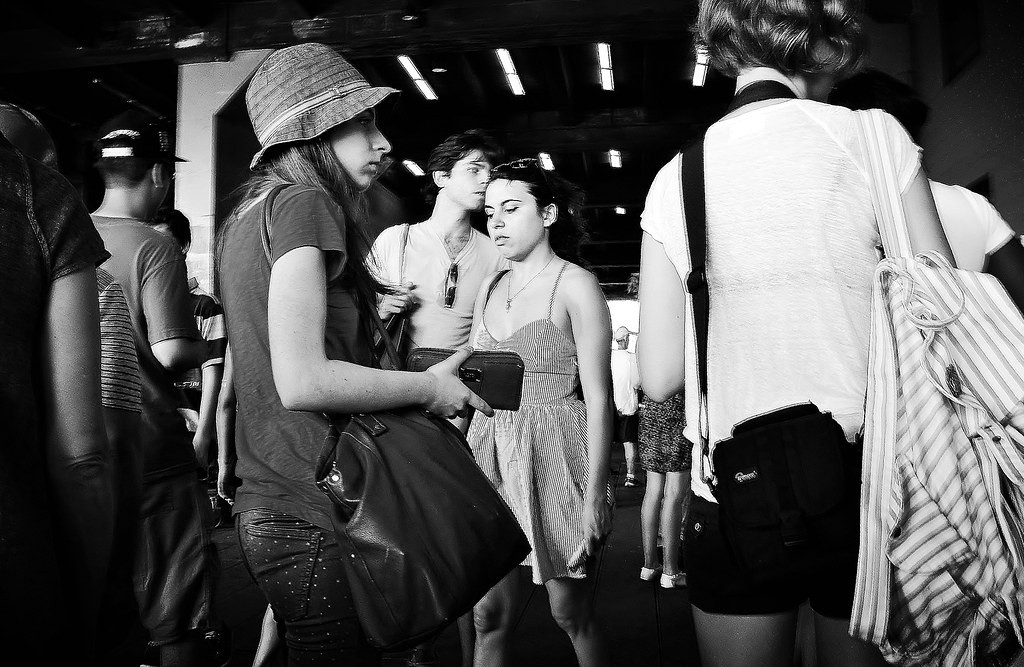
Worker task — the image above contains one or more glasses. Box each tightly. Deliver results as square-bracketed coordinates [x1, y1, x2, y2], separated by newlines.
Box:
[444, 263, 458, 309]
[490, 157, 549, 184]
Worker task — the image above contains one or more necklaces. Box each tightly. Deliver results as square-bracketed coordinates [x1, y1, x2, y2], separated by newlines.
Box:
[506, 253, 555, 313]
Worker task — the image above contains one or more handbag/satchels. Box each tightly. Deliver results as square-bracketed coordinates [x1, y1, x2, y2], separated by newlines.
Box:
[848, 105, 1024, 667]
[312, 409, 532, 659]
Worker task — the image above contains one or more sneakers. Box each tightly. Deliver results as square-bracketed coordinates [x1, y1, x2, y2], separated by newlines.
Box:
[639, 564, 663, 581]
[660, 571, 687, 588]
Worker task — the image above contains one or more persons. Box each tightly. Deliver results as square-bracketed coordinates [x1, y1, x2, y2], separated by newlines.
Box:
[636, 0, 958, 667]
[445, 158, 613, 667]
[86, 114, 279, 667]
[0, 104, 143, 667]
[365, 130, 508, 433]
[640, 395, 694, 588]
[611, 326, 642, 486]
[831, 66, 1024, 315]
[216, 43, 495, 667]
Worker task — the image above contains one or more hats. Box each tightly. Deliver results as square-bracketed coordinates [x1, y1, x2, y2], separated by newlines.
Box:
[244, 42, 404, 172]
[615, 326, 634, 340]
[96, 116, 189, 166]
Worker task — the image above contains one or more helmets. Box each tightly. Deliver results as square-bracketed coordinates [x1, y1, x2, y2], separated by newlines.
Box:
[0, 104, 58, 169]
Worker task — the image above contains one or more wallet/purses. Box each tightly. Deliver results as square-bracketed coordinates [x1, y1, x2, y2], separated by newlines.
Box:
[406, 348, 524, 411]
